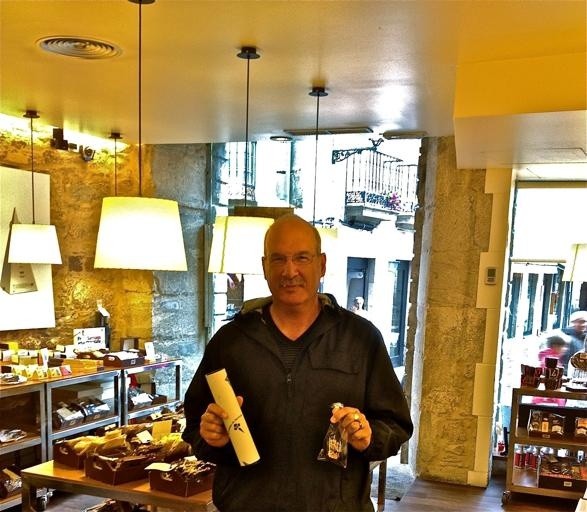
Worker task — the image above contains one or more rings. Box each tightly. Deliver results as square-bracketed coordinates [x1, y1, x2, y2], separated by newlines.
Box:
[353, 413, 360, 421]
[354, 420, 362, 429]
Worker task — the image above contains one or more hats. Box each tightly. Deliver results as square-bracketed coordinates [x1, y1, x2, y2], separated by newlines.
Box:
[571, 311, 587, 322]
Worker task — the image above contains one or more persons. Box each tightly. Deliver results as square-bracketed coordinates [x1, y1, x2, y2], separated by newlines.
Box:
[180, 213, 413, 512]
[560, 310, 587, 375]
[346, 296, 369, 320]
[532, 329, 567, 406]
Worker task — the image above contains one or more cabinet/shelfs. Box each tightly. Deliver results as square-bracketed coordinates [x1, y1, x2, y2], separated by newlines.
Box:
[502, 381, 587, 505]
[26, 366, 121, 492]
[98, 350, 181, 427]
[0, 380, 47, 512]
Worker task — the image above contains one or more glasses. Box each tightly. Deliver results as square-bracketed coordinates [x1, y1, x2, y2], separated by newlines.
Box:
[262, 253, 321, 265]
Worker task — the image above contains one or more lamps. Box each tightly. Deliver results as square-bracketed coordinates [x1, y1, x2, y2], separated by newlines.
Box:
[207, 46, 275, 275]
[7, 110, 62, 265]
[308, 87, 339, 258]
[562, 244, 587, 282]
[94, 1, 188, 271]
